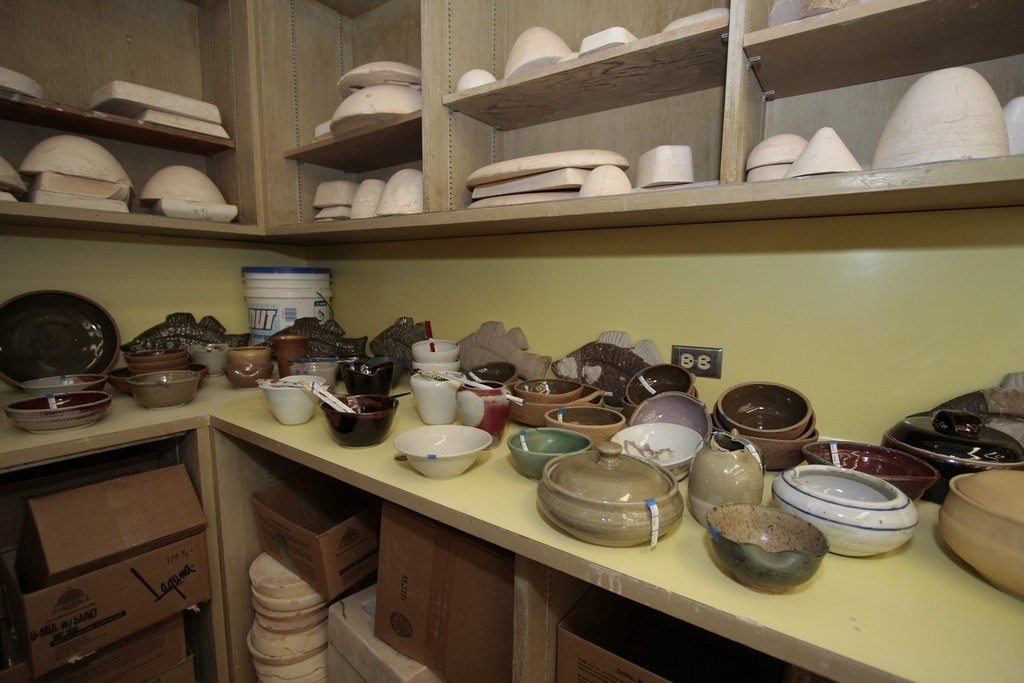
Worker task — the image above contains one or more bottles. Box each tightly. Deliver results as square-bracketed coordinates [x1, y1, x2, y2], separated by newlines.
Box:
[686, 427, 766, 530]
[456, 381, 512, 451]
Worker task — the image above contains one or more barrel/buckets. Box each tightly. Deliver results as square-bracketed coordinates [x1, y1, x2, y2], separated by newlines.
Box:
[240, 265, 335, 346]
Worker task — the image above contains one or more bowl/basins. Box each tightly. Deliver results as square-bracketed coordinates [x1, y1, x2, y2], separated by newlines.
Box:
[0, 289, 1024, 604]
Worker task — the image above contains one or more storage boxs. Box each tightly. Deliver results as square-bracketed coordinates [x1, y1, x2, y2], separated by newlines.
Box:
[0, 459, 814, 683]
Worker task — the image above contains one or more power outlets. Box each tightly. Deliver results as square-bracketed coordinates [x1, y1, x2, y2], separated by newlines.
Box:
[670, 345, 723, 378]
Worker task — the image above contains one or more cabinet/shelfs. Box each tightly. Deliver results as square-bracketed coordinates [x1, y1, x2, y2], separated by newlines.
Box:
[208, 366, 1024, 683]
[0, 0, 1024, 246]
[0, 386, 230, 683]
